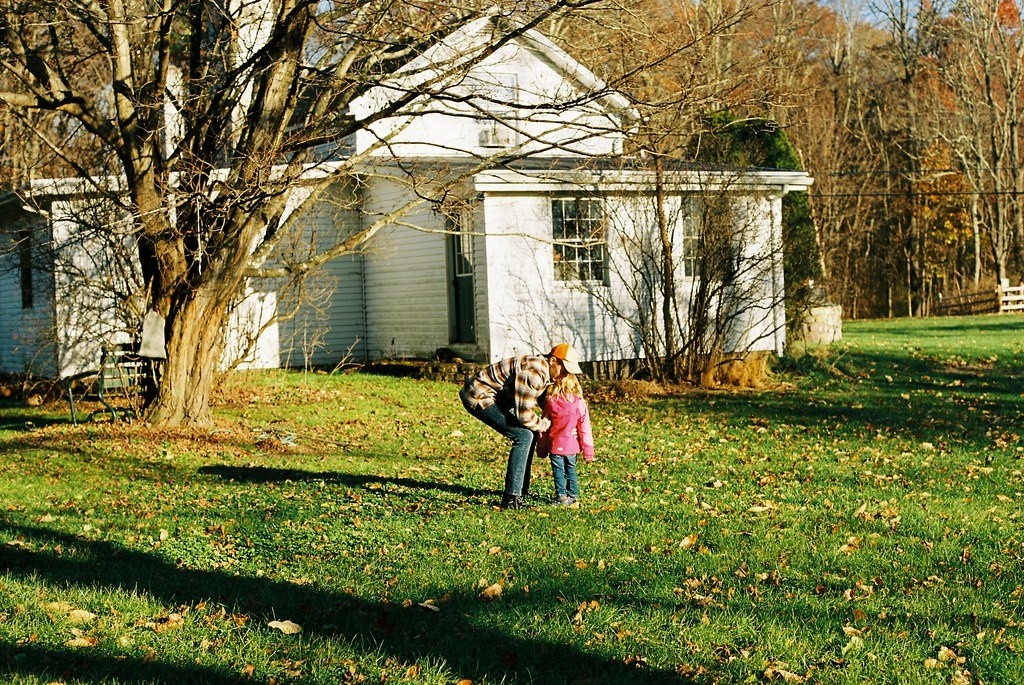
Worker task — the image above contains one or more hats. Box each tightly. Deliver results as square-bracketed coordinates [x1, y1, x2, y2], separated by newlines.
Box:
[551, 343, 583, 374]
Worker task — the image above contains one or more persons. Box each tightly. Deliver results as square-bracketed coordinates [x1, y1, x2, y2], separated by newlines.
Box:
[459, 343, 583, 509]
[536, 373, 594, 505]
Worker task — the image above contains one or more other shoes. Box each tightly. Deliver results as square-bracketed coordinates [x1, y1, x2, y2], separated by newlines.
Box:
[547, 494, 568, 509]
[500, 490, 540, 512]
[567, 496, 579, 509]
